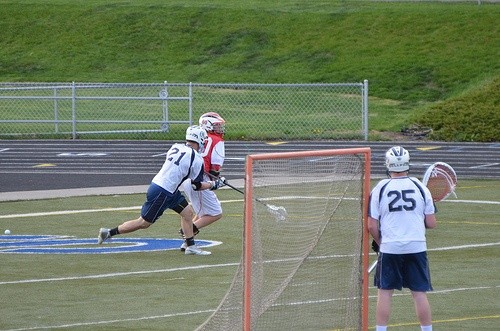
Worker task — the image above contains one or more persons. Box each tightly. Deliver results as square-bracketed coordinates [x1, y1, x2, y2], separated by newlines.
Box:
[180, 112, 225, 251]
[368, 146, 439, 331]
[97, 124, 228, 256]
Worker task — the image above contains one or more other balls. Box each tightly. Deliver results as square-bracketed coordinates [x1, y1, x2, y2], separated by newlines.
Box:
[4, 229, 11, 235]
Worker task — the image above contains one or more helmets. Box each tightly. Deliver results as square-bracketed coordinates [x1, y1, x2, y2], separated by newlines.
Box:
[198, 111, 225, 133]
[385, 146, 409, 172]
[186, 124, 208, 153]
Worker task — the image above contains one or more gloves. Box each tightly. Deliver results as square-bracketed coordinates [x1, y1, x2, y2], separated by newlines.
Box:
[371, 240, 379, 253]
[209, 169, 221, 181]
[208, 177, 226, 191]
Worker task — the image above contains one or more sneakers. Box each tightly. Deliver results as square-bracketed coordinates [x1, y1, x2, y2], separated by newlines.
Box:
[185, 247, 212, 256]
[98, 228, 111, 245]
[181, 243, 187, 250]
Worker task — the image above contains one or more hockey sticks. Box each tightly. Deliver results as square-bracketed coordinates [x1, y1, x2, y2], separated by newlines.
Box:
[367, 161, 458, 276]
[202, 169, 288, 222]
